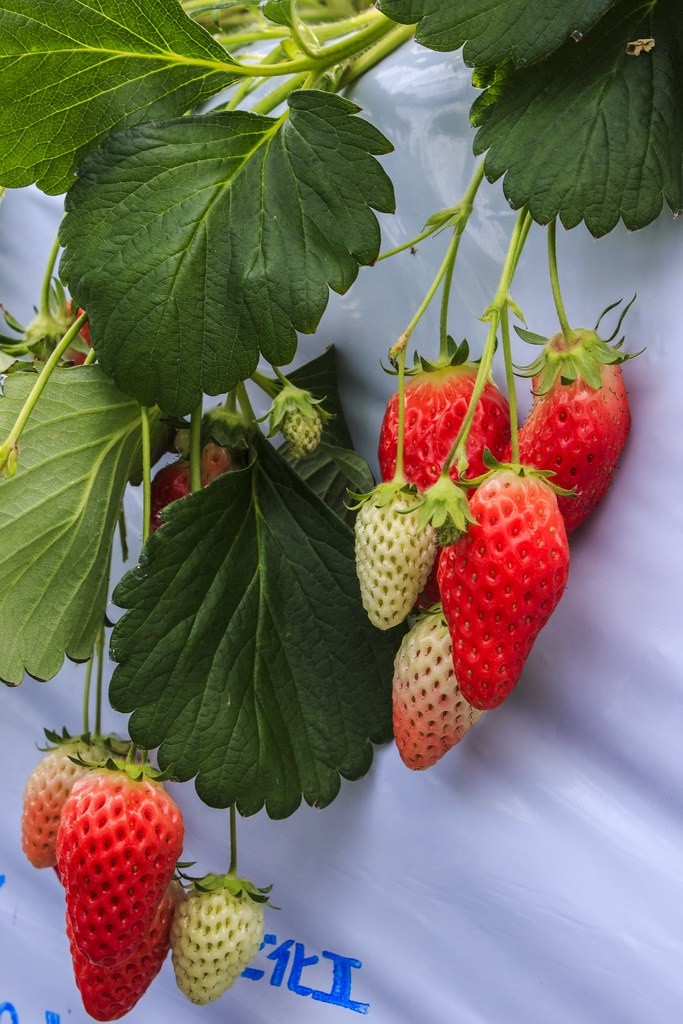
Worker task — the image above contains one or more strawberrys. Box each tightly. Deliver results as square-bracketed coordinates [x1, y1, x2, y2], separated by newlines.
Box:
[21, 301, 630, 1021]
